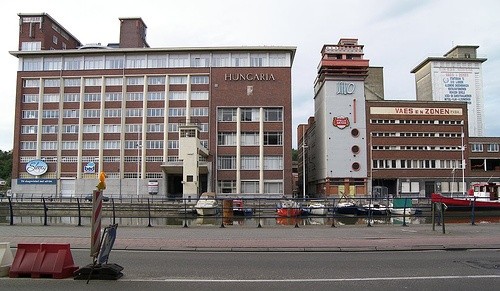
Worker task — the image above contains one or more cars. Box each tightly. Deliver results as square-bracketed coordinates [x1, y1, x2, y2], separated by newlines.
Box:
[85, 194, 109, 202]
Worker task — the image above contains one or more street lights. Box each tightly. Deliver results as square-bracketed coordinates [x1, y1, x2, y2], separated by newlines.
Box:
[301, 144, 308, 201]
[135, 143, 142, 196]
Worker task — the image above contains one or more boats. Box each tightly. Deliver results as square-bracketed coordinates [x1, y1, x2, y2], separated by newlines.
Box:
[307, 199, 329, 216]
[276, 200, 302, 217]
[231, 199, 252, 216]
[389, 202, 416, 216]
[362, 202, 387, 216]
[194, 191, 219, 216]
[334, 202, 359, 215]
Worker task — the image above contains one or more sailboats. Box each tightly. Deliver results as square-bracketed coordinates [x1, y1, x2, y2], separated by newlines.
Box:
[431, 137, 500, 210]
